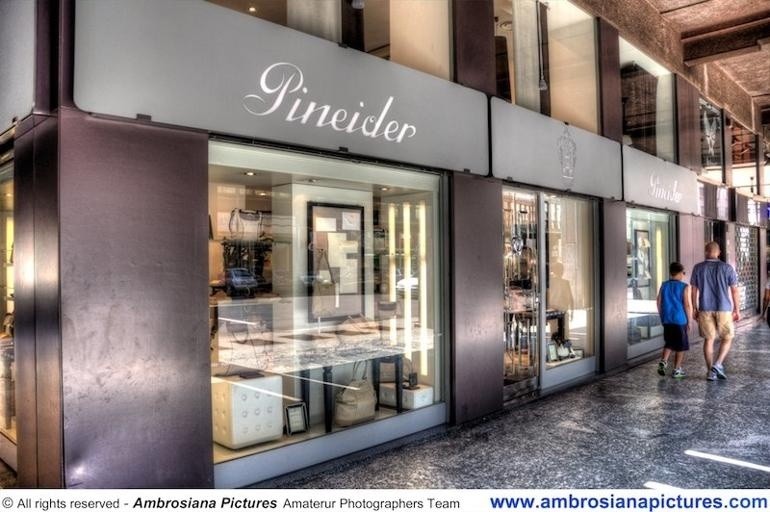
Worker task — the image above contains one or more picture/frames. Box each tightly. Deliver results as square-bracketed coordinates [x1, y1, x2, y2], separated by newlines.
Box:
[307, 200, 365, 324]
[284, 402, 310, 437]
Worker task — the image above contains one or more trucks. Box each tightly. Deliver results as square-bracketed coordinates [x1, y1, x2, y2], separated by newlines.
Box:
[627, 254, 650, 288]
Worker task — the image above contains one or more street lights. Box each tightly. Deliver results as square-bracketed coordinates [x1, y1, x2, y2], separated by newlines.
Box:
[503, 191, 530, 240]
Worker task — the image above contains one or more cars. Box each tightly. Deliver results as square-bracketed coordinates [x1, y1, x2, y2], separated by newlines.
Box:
[303, 268, 421, 300]
[504, 285, 533, 297]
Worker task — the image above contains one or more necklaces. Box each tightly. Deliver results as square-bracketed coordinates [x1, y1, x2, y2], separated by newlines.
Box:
[226, 320, 250, 344]
[348, 312, 371, 335]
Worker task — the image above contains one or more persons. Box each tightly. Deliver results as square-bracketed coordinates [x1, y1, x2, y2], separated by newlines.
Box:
[754, 269, 770, 330]
[542, 261, 581, 348]
[656, 262, 694, 380]
[689, 241, 741, 382]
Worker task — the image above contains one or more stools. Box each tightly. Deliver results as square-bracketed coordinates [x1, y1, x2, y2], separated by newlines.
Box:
[211, 371, 284, 450]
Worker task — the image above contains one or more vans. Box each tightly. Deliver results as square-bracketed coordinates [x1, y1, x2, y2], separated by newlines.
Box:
[223, 266, 259, 299]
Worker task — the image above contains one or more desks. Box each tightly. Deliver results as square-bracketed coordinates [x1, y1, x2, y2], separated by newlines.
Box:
[219, 327, 435, 435]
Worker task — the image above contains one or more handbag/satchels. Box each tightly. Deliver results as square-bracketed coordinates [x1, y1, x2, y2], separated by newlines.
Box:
[229, 208, 264, 240]
[210, 321, 268, 376]
[225, 267, 258, 297]
[336, 314, 381, 347]
[380, 357, 414, 383]
[334, 378, 377, 427]
[311, 280, 339, 319]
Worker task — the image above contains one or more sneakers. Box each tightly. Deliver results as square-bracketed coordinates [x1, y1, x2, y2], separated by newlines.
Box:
[707, 365, 727, 381]
[657, 359, 666, 376]
[671, 367, 689, 378]
